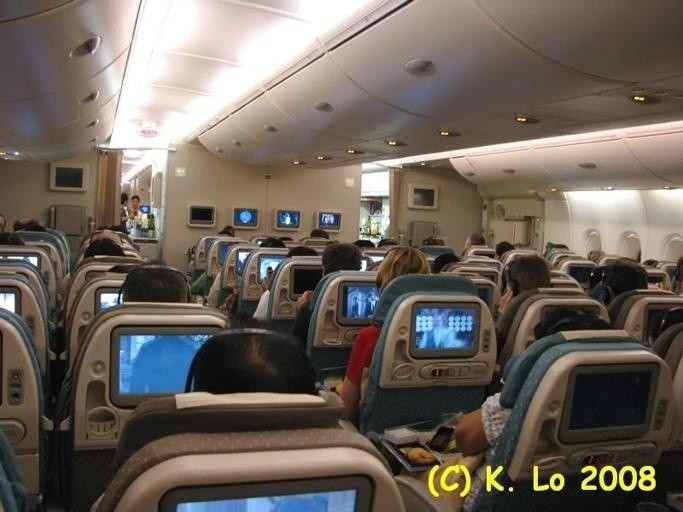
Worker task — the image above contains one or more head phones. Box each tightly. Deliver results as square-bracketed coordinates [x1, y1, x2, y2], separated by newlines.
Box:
[592, 256, 637, 306]
[501, 259, 554, 297]
[670, 260, 682, 294]
[185, 328, 325, 393]
[118, 264, 191, 303]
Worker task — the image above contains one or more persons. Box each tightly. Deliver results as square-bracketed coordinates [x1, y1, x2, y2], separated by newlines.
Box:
[0, 429, 29, 512]
[643, 258, 656, 266]
[659, 306, 683, 331]
[423, 234, 445, 247]
[430, 252, 459, 273]
[13, 216, 38, 231]
[220, 238, 285, 318]
[251, 246, 319, 322]
[671, 254, 683, 294]
[464, 234, 485, 249]
[588, 249, 604, 263]
[281, 243, 362, 343]
[335, 246, 430, 422]
[190, 271, 205, 295]
[130, 195, 140, 215]
[0, 231, 26, 246]
[493, 255, 551, 323]
[349, 296, 359, 316]
[225, 225, 235, 235]
[454, 313, 613, 512]
[194, 329, 316, 395]
[123, 260, 188, 303]
[357, 307, 363, 309]
[495, 239, 514, 257]
[378, 238, 398, 248]
[365, 294, 378, 316]
[599, 257, 648, 307]
[83, 239, 125, 258]
[121, 193, 130, 224]
[354, 240, 375, 247]
[311, 229, 329, 240]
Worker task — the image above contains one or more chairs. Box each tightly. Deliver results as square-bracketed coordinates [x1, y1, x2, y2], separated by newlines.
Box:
[0, 273, 55, 386]
[606, 288, 683, 347]
[90, 390, 407, 512]
[249, 236, 267, 245]
[25, 240, 65, 284]
[47, 302, 228, 512]
[60, 276, 125, 370]
[314, 273, 501, 475]
[393, 330, 676, 512]
[299, 238, 330, 245]
[311, 246, 325, 255]
[283, 240, 303, 248]
[0, 245, 62, 306]
[14, 231, 67, 277]
[186, 235, 216, 295]
[482, 288, 611, 403]
[194, 239, 250, 304]
[0, 308, 55, 512]
[651, 322, 683, 512]
[72, 229, 142, 265]
[304, 269, 381, 394]
[358, 245, 683, 290]
[0, 259, 56, 327]
[235, 247, 290, 321]
[269, 256, 325, 321]
[45, 228, 70, 275]
[60, 255, 145, 323]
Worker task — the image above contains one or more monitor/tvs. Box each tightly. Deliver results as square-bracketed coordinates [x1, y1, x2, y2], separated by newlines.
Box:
[290, 266, 324, 300]
[159, 477, 373, 511]
[232, 206, 260, 229]
[110, 327, 222, 406]
[558, 364, 658, 445]
[257, 255, 288, 285]
[567, 265, 593, 286]
[409, 303, 481, 359]
[188, 204, 217, 228]
[218, 242, 230, 265]
[338, 283, 381, 327]
[0, 286, 20, 318]
[273, 208, 303, 231]
[409, 185, 437, 210]
[140, 204, 149, 213]
[96, 286, 122, 313]
[1, 254, 40, 269]
[317, 210, 343, 232]
[54, 167, 82, 188]
[235, 249, 251, 276]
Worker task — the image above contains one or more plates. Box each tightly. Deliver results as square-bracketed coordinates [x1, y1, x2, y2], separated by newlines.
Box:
[375, 415, 465, 473]
[324, 374, 348, 398]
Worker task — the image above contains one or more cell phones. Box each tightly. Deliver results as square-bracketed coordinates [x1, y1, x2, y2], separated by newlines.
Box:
[429, 426, 455, 452]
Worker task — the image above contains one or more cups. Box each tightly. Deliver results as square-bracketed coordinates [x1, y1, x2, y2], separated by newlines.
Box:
[443, 409, 465, 431]
